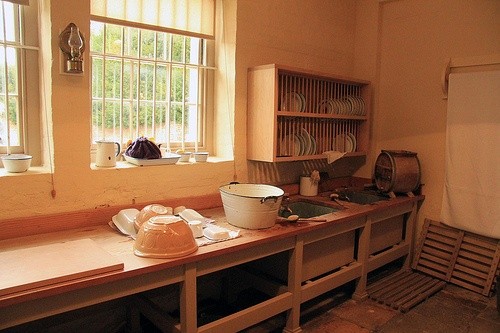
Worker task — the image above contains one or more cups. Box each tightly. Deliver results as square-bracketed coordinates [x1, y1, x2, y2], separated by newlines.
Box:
[96, 140, 120, 167]
[299, 174, 318, 196]
[188, 220, 204, 238]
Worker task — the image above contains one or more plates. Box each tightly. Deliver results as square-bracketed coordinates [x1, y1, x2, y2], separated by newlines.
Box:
[281, 127, 317, 157]
[332, 131, 356, 153]
[280, 92, 306, 119]
[316, 95, 367, 122]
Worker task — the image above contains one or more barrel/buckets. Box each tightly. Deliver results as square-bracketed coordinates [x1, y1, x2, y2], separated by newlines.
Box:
[219, 180, 285, 230]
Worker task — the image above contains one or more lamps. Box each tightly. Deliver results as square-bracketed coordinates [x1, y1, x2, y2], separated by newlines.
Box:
[58, 23, 88, 72]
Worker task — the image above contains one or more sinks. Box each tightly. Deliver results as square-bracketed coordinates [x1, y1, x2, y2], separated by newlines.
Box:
[277, 198, 349, 219]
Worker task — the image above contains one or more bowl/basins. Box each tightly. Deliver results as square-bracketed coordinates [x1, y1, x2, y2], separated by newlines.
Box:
[111, 207, 140, 239]
[0, 154, 32, 173]
[178, 152, 192, 162]
[132, 215, 199, 259]
[166, 206, 232, 239]
[193, 153, 208, 162]
[133, 204, 170, 230]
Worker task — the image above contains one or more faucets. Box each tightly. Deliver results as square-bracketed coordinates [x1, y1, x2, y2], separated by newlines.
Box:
[282, 196, 290, 202]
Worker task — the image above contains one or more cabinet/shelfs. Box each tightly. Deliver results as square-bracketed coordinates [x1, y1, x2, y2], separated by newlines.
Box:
[248, 63, 371, 162]
